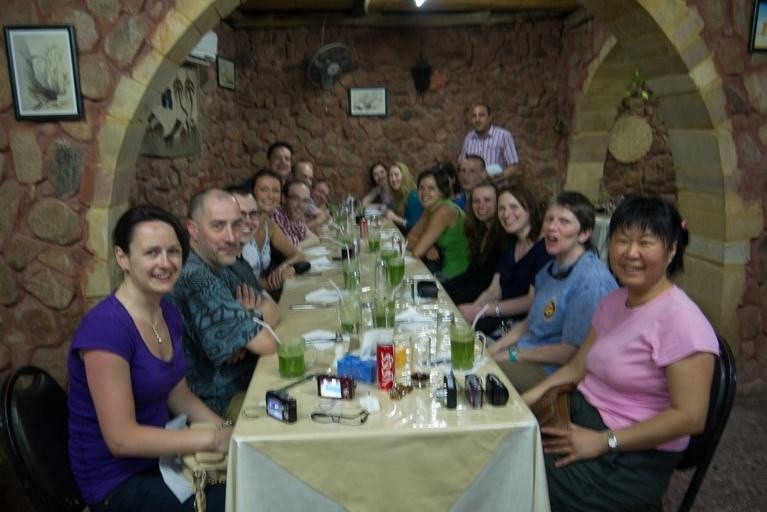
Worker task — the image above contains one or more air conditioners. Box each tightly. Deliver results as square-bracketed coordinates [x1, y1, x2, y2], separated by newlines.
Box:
[185, 28, 219, 67]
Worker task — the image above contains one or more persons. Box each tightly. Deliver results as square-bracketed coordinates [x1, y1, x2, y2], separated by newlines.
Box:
[362, 154, 619, 421]
[167, 143, 330, 420]
[66, 203, 235, 511]
[457, 104, 519, 191]
[519, 194, 721, 512]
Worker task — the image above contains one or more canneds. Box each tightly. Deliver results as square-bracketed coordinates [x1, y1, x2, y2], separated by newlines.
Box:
[377, 344, 394, 390]
[359, 217, 368, 238]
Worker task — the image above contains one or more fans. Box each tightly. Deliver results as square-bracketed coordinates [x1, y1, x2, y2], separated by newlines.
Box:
[307, 42, 352, 91]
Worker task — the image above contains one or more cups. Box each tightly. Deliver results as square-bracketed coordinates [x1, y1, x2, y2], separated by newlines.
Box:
[276, 194, 486, 380]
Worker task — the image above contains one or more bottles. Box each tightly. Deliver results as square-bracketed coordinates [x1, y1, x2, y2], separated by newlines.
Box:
[391, 308, 455, 392]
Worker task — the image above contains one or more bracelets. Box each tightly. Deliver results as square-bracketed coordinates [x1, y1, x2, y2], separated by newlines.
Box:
[217, 419, 232, 434]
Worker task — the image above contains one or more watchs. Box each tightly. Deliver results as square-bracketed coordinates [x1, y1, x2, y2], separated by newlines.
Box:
[607, 429, 618, 455]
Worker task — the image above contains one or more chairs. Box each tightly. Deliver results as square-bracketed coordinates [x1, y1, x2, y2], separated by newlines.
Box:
[624, 335, 738, 512]
[1, 361, 102, 512]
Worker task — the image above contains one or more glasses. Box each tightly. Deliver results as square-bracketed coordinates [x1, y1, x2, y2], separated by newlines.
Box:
[240, 209, 259, 223]
[292, 196, 311, 205]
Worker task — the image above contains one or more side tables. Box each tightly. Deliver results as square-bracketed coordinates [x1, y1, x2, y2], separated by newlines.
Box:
[589, 212, 617, 262]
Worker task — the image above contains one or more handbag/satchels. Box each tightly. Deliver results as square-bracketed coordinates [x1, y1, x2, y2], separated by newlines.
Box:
[179, 419, 233, 512]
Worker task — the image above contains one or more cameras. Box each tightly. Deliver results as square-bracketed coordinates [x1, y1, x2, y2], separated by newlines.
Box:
[317, 375, 354, 401]
[465, 375, 483, 409]
[265, 390, 297, 423]
[418, 281, 438, 299]
[438, 374, 457, 409]
[486, 374, 509, 405]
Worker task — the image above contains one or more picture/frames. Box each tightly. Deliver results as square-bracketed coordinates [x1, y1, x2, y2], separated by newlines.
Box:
[2, 23, 86, 121]
[347, 86, 389, 118]
[216, 54, 237, 91]
[748, 1, 767, 55]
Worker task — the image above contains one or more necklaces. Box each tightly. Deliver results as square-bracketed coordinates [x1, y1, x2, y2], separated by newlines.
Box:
[151, 321, 162, 343]
